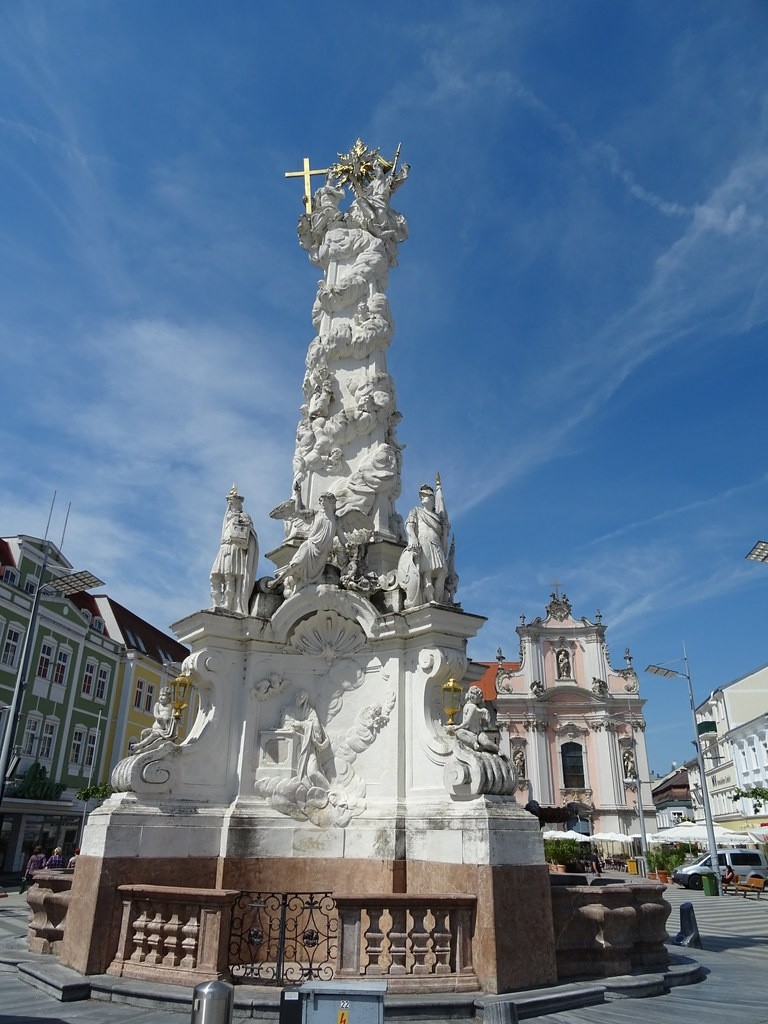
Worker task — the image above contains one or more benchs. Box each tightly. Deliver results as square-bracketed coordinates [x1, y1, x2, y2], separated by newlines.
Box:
[722, 874, 742, 893]
[736, 878, 765, 900]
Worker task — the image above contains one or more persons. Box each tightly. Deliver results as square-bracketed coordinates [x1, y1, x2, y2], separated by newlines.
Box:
[623, 753, 636, 779]
[405, 472, 459, 606]
[558, 651, 571, 679]
[550, 592, 569, 605]
[256, 672, 282, 695]
[514, 752, 525, 775]
[269, 138, 406, 588]
[46, 847, 63, 869]
[67, 848, 80, 868]
[590, 850, 630, 878]
[131, 686, 176, 754]
[279, 689, 330, 791]
[210, 481, 259, 616]
[324, 691, 396, 787]
[721, 864, 735, 884]
[453, 685, 500, 754]
[19, 846, 46, 894]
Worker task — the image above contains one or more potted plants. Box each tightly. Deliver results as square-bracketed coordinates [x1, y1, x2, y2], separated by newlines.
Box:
[543, 837, 582, 872]
[644, 846, 674, 884]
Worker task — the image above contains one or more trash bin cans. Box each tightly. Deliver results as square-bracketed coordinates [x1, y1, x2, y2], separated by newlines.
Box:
[702, 872, 719, 897]
[627, 858, 637, 875]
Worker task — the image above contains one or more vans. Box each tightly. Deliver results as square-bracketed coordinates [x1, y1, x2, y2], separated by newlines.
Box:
[670, 848, 768, 891]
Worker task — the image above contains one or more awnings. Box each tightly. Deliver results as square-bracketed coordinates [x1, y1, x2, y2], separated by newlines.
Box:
[542, 820, 768, 864]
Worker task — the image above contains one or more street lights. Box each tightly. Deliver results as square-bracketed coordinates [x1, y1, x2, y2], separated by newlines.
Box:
[642, 638, 724, 897]
[1, 541, 105, 805]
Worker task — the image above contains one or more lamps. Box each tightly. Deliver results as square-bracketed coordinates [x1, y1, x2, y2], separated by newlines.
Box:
[441, 678, 464, 725]
[170, 673, 189, 719]
